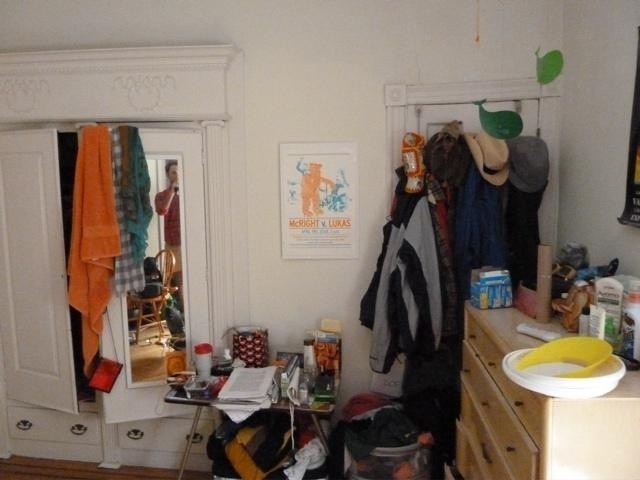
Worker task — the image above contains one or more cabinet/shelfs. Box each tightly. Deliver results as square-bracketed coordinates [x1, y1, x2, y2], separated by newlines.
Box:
[0, 120, 229, 474]
[453, 298, 640, 480]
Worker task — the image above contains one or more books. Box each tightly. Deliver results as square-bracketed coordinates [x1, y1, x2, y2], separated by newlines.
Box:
[210, 365, 277, 411]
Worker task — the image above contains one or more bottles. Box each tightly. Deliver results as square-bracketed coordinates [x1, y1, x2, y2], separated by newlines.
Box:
[621, 292, 639, 364]
[299, 370, 315, 406]
[302, 340, 316, 371]
[220, 347, 232, 360]
[579, 304, 592, 336]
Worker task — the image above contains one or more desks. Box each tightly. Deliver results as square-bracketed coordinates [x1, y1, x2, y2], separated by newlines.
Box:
[163, 387, 336, 480]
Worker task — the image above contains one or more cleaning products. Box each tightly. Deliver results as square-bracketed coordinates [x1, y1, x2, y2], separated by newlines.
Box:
[621, 279, 640, 363]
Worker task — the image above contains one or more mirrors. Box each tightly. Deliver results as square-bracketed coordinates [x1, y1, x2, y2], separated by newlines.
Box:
[120, 151, 192, 390]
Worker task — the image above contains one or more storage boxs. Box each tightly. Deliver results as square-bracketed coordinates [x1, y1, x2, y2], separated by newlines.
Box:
[470, 267, 513, 309]
[225, 425, 292, 480]
[351, 442, 434, 480]
[233, 331, 269, 368]
[313, 338, 342, 379]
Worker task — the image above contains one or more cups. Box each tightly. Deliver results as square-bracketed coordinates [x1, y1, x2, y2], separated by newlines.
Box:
[173, 184, 180, 198]
[480, 287, 489, 309]
[193, 343, 213, 377]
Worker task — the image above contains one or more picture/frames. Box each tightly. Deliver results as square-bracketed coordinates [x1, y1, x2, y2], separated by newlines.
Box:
[279, 141, 360, 260]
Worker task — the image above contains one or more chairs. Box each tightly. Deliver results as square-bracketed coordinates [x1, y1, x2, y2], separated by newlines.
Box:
[131, 247, 176, 345]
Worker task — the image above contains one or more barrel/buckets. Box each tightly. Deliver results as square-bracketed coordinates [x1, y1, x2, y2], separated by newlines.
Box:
[348, 404, 433, 480]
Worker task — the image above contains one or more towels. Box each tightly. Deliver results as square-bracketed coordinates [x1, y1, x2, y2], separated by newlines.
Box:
[65, 125, 124, 379]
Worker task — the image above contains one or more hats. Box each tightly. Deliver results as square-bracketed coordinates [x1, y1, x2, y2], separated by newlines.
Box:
[463, 129, 510, 186]
[422, 131, 462, 180]
[508, 135, 550, 193]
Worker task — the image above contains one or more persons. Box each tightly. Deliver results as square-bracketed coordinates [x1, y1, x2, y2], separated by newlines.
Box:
[155, 159, 182, 278]
